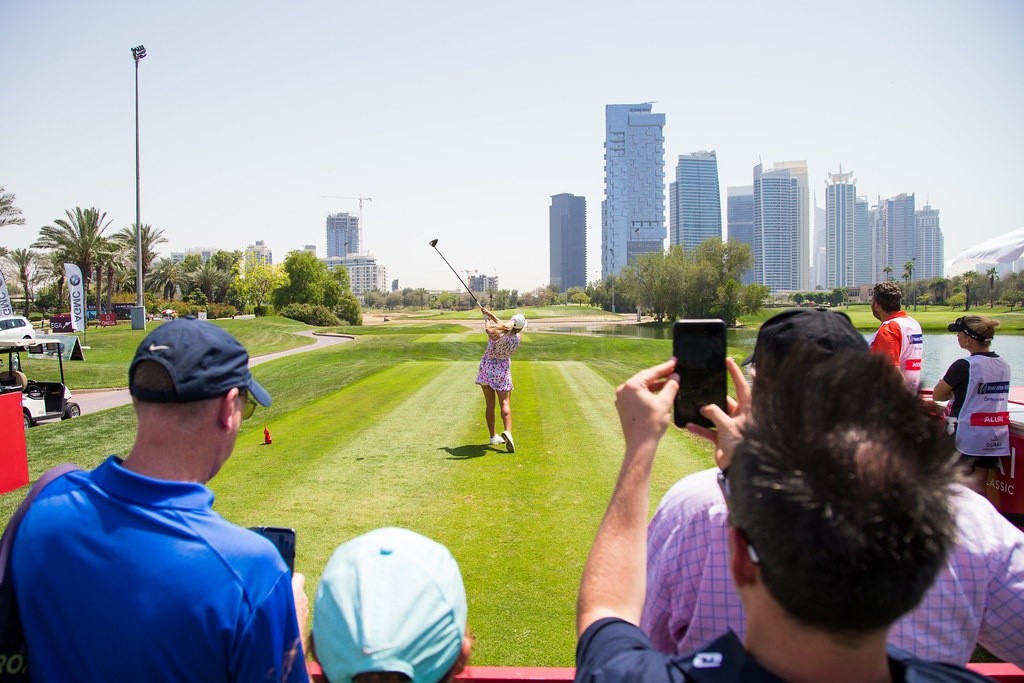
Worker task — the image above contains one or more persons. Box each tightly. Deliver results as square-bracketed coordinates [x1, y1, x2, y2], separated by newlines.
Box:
[868, 282, 924, 397]
[9, 316, 313, 683]
[642, 307, 1024, 671]
[304, 526, 471, 683]
[933, 315, 1011, 495]
[475, 307, 526, 453]
[575, 334, 996, 683]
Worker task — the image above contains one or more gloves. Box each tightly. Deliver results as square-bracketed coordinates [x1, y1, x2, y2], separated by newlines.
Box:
[482, 312, 491, 320]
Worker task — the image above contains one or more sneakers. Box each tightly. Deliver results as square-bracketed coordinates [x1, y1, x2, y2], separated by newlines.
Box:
[501, 431, 515, 453]
[490, 433, 505, 445]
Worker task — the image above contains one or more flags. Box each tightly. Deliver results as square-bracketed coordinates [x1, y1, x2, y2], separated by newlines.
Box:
[63, 262, 85, 333]
[0, 270, 14, 318]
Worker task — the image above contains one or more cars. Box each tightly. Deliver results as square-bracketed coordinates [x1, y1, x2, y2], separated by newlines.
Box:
[818, 302, 831, 308]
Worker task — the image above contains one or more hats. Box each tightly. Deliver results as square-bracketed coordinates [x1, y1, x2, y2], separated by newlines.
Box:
[127, 315, 272, 408]
[510, 314, 527, 329]
[741, 307, 855, 366]
[948, 316, 994, 342]
[313, 526, 468, 683]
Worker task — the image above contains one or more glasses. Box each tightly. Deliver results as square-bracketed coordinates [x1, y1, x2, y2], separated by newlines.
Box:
[238, 393, 257, 420]
[716, 465, 759, 564]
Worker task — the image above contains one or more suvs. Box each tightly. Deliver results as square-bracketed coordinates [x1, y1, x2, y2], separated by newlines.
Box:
[0, 315, 36, 351]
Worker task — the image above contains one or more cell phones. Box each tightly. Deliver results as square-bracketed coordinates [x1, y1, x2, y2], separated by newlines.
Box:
[669, 319, 729, 428]
[248, 525, 294, 581]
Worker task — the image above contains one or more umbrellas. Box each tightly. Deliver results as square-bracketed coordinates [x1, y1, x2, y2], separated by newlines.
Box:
[161, 309, 175, 318]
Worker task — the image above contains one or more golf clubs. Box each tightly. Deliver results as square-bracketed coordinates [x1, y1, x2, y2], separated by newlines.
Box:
[429, 238, 485, 315]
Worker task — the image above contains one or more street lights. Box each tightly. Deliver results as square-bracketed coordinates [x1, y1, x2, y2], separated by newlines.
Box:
[130, 45, 148, 330]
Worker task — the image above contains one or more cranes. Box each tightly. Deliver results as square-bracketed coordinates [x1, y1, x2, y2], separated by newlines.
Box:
[323, 193, 373, 254]
[457, 266, 478, 291]
